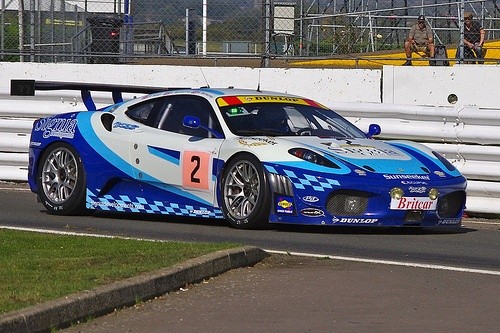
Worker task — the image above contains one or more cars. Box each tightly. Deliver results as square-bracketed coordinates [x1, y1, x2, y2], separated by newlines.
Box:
[10, 77, 468, 233]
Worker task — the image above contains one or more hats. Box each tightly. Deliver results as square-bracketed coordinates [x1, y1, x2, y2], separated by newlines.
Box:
[464, 12, 473, 20]
[418, 15, 425, 21]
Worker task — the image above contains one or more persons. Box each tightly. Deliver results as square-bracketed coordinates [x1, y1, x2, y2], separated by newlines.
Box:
[264, 108, 312, 136]
[456, 13, 485, 64]
[402, 15, 436, 66]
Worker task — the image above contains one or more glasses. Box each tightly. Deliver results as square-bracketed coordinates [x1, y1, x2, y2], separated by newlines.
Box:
[464, 17, 469, 20]
[418, 21, 425, 24]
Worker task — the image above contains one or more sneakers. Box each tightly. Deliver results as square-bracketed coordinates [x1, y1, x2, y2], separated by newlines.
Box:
[402, 61, 412, 66]
[429, 60, 436, 66]
